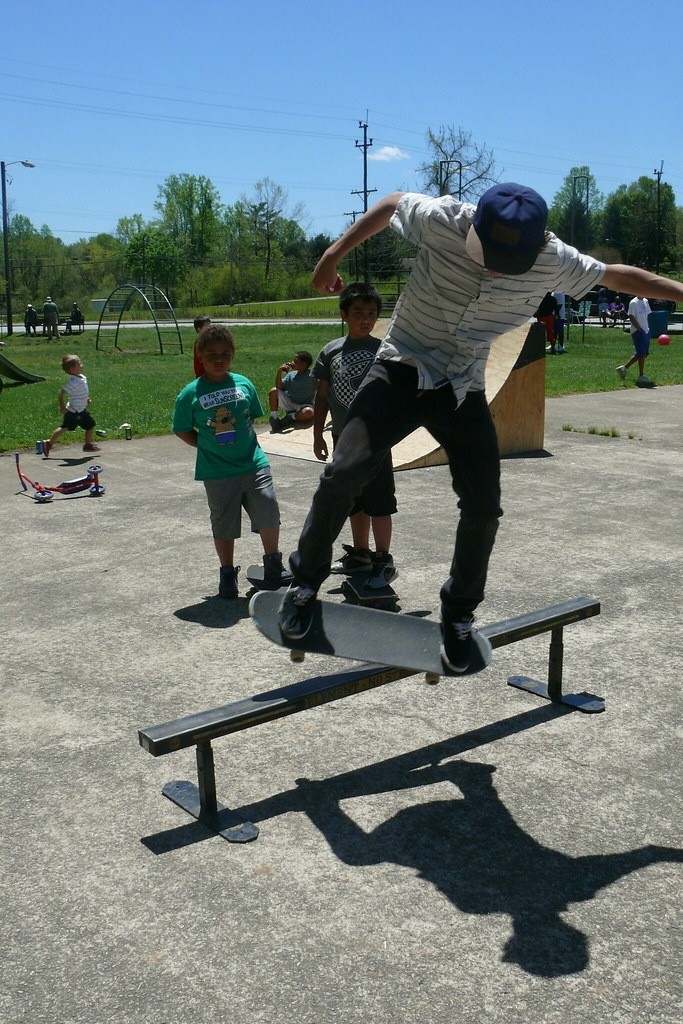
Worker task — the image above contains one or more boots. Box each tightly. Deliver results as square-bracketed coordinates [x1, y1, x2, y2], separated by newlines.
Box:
[217, 567, 239, 599]
[263, 552, 294, 582]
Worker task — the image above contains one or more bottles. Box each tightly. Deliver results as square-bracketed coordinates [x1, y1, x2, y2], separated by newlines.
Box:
[95, 429, 106, 436]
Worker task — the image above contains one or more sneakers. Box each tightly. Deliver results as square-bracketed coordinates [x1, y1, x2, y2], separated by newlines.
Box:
[437, 602, 477, 673]
[277, 582, 320, 639]
[366, 552, 399, 588]
[331, 543, 374, 574]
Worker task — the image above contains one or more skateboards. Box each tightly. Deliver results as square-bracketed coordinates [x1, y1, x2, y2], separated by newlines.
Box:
[245, 563, 289, 600]
[344, 576, 401, 610]
[249, 589, 493, 685]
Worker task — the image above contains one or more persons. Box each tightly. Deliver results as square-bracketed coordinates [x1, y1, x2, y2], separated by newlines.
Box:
[269, 351, 315, 434]
[597, 286, 625, 328]
[314, 284, 398, 576]
[24, 303, 38, 337]
[42, 297, 61, 340]
[194, 316, 211, 376]
[277, 182, 683, 671]
[173, 325, 295, 597]
[63, 302, 81, 335]
[42, 355, 101, 457]
[546, 289, 565, 351]
[615, 296, 652, 383]
[533, 291, 560, 353]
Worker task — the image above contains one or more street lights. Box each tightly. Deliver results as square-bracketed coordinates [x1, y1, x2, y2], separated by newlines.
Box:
[141, 225, 159, 310]
[1, 160, 35, 335]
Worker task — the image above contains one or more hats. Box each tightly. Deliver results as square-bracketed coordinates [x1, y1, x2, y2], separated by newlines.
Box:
[47, 297, 52, 302]
[466, 182, 550, 275]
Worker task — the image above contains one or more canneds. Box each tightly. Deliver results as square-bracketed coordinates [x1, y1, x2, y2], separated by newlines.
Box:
[94, 430, 106, 436]
[125, 428, 132, 440]
[35, 440, 42, 454]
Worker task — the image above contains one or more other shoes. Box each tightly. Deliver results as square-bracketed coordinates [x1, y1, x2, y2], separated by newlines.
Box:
[617, 366, 626, 380]
[42, 439, 52, 457]
[558, 346, 565, 352]
[545, 346, 555, 352]
[82, 443, 101, 451]
[270, 417, 281, 432]
[281, 411, 295, 423]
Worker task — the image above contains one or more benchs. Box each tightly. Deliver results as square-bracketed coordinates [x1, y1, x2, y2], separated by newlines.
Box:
[30, 315, 84, 333]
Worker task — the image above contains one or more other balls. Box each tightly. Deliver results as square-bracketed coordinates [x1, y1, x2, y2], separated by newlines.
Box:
[658, 334, 670, 345]
